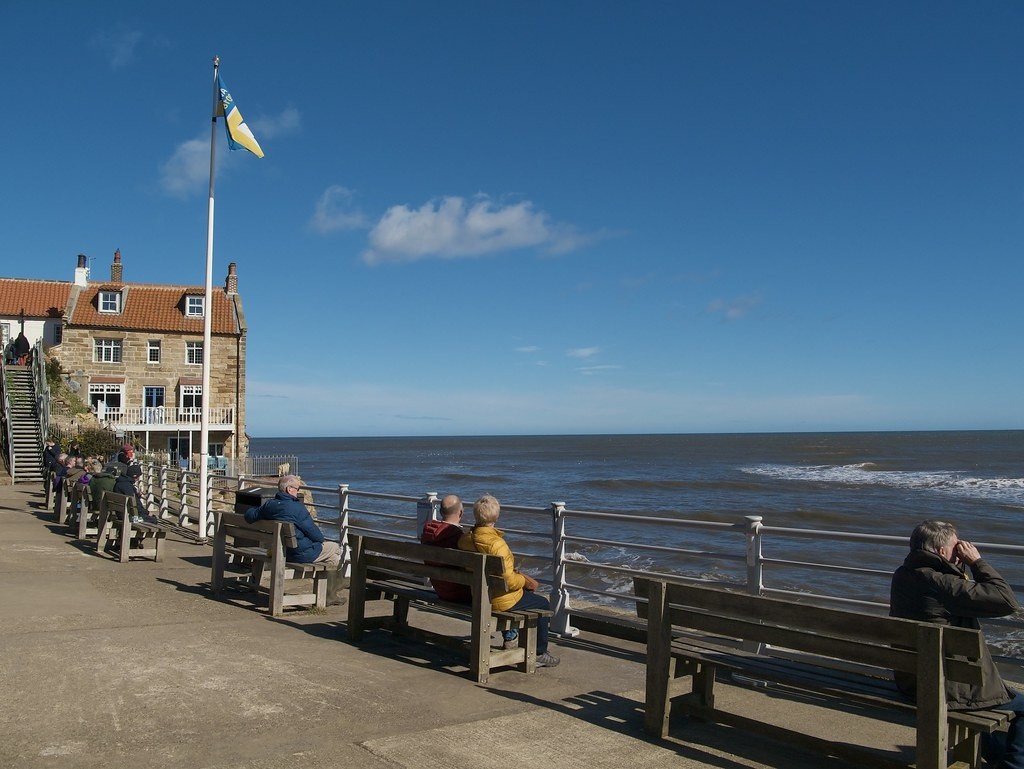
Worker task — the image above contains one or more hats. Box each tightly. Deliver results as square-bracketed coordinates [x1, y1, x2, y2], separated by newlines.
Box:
[124, 444, 132, 450]
[126, 465, 142, 478]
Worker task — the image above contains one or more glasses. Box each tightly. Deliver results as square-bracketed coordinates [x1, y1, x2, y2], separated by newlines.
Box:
[289, 485, 299, 492]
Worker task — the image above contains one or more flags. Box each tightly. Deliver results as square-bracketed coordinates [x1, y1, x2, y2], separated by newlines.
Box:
[212, 68, 264, 159]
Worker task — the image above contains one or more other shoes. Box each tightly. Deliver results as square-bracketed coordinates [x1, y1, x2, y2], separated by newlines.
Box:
[327, 570, 351, 605]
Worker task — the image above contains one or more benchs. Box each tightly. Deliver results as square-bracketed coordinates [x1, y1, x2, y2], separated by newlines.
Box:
[69, 481, 114, 541]
[96, 490, 167, 562]
[345, 532, 554, 685]
[210, 511, 337, 617]
[44, 470, 57, 511]
[54, 476, 81, 524]
[633, 574, 1024, 769]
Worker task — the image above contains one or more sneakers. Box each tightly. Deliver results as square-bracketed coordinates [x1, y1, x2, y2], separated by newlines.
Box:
[503, 636, 518, 649]
[536, 654, 560, 669]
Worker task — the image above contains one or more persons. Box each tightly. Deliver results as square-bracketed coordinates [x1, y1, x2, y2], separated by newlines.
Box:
[4, 337, 14, 367]
[243, 473, 351, 607]
[68, 441, 81, 457]
[457, 494, 562, 670]
[113, 464, 158, 550]
[89, 467, 118, 512]
[9, 340, 18, 365]
[43, 437, 63, 506]
[110, 443, 134, 465]
[887, 518, 1024, 769]
[13, 333, 29, 366]
[51, 452, 105, 487]
[420, 495, 524, 653]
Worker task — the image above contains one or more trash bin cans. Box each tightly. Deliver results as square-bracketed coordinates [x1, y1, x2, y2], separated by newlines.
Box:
[231, 486, 306, 573]
